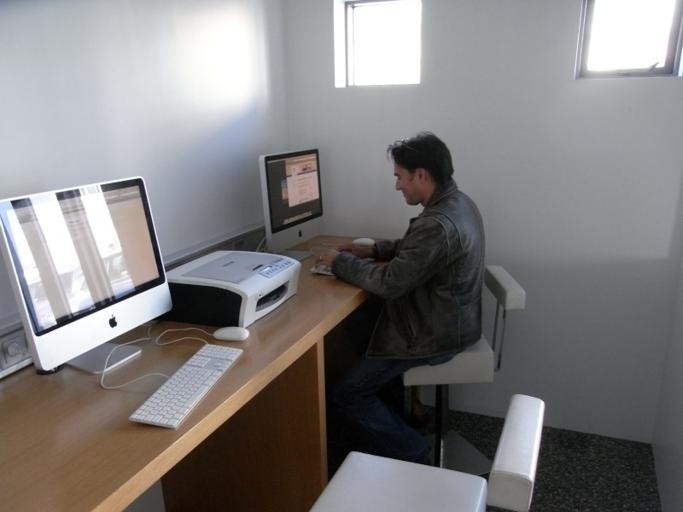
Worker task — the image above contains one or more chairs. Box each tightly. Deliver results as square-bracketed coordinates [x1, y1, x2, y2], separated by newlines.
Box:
[309, 394, 545, 512]
[386, 265, 527, 476]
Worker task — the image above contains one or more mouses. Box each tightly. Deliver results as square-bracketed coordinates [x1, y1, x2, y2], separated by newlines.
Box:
[352, 238, 374, 245]
[213, 326, 249, 340]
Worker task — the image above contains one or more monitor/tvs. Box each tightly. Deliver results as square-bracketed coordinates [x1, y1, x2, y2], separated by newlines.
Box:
[0, 175, 175, 374]
[258, 148, 335, 261]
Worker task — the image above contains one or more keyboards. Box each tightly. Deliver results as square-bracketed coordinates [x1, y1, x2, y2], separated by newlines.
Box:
[128, 344, 243, 428]
[310, 247, 376, 275]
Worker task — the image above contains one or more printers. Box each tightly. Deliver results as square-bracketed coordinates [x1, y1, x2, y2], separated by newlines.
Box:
[153, 250, 301, 328]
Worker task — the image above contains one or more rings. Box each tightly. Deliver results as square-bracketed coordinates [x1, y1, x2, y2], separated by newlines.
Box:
[319, 255, 322, 261]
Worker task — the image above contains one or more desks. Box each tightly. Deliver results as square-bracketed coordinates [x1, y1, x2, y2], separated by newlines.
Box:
[1, 235, 387, 512]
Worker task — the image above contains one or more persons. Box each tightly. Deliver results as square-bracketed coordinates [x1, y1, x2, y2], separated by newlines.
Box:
[314, 131, 485, 466]
